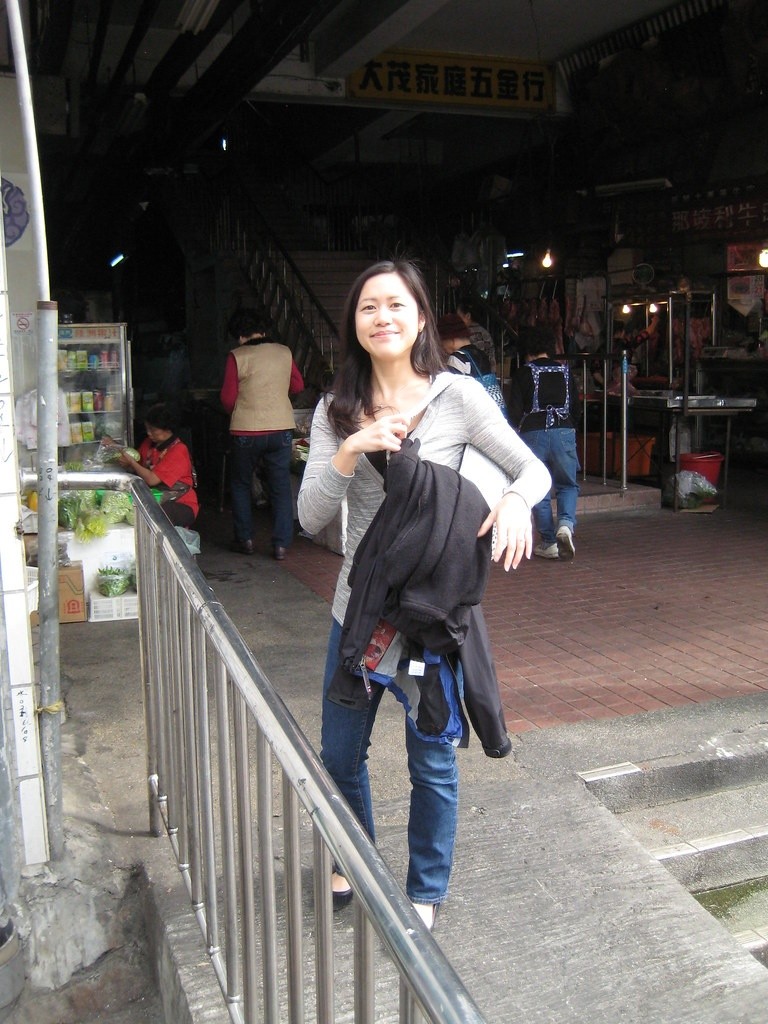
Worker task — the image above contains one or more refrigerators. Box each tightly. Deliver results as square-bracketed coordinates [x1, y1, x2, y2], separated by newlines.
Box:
[54, 321, 135, 468]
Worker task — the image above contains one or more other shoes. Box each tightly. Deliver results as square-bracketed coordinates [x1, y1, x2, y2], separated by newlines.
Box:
[229, 539, 253, 554]
[273, 545, 288, 559]
[429, 903, 440, 932]
[327, 870, 353, 911]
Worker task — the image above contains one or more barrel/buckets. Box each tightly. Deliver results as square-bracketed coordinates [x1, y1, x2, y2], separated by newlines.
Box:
[672, 451, 725, 503]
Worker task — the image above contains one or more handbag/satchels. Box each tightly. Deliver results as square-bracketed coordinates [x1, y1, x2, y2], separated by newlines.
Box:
[149, 481, 190, 503]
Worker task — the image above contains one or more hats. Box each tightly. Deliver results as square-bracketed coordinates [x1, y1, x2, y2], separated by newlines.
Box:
[436, 314, 473, 340]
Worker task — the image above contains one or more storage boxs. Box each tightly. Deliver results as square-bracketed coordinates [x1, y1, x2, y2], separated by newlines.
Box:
[88, 588, 139, 622]
[576, 431, 657, 478]
[27, 566, 39, 615]
[58, 560, 88, 624]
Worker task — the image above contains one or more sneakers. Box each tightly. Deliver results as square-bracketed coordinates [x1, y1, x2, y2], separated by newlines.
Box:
[554, 526, 576, 559]
[534, 541, 559, 559]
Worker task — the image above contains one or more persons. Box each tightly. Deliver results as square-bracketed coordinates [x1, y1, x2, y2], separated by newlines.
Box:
[297, 256, 552, 931]
[508, 340, 579, 561]
[118, 407, 201, 530]
[220, 310, 305, 560]
[591, 314, 661, 386]
[457, 300, 497, 373]
[434, 312, 508, 422]
